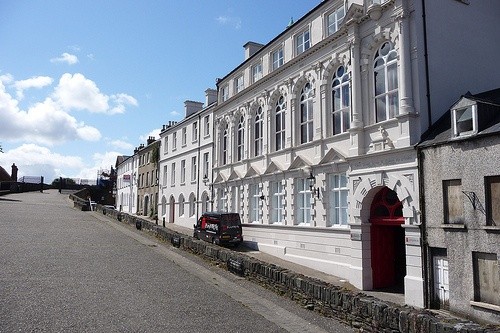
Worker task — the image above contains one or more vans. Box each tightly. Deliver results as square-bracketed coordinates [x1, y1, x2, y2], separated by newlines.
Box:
[193, 211, 243, 247]
